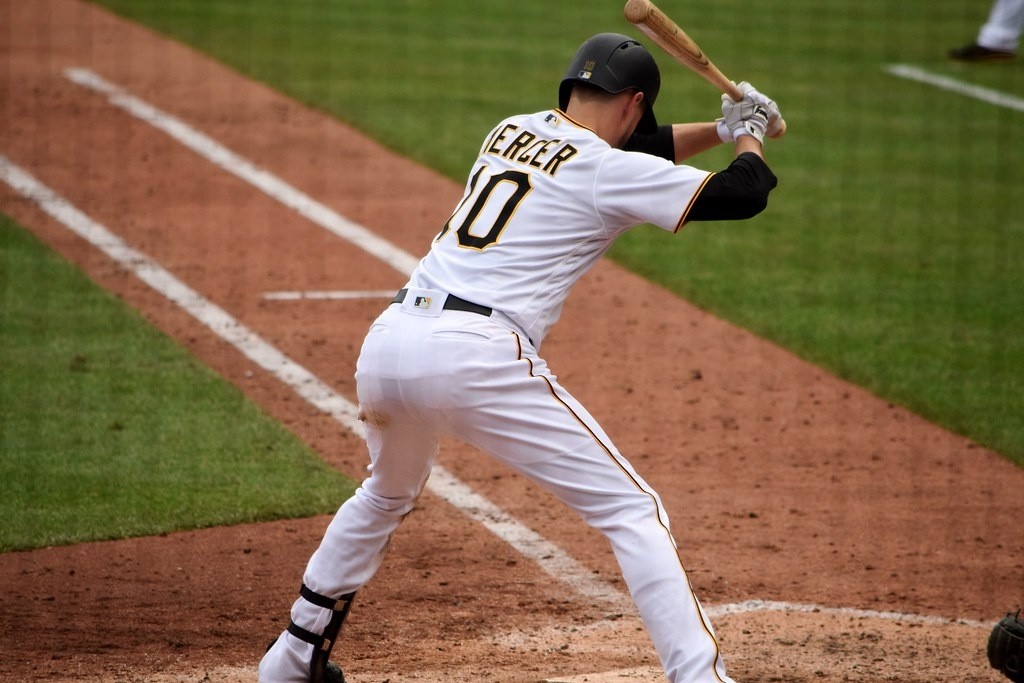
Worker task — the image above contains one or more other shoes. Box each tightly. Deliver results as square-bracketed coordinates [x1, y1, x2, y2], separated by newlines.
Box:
[310, 661, 343, 683]
[946, 42, 1017, 63]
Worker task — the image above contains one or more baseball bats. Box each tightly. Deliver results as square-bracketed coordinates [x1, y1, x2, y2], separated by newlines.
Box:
[623, 0, 787, 141]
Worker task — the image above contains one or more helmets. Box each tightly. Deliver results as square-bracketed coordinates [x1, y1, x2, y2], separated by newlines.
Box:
[558, 32, 661, 137]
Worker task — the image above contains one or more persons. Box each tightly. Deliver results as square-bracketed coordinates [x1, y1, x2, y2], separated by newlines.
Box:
[950, 0, 1024, 62]
[258, 32, 782, 683]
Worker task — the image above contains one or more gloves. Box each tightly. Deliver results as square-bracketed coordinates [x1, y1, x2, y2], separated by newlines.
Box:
[721, 81, 770, 148]
[714, 98, 782, 143]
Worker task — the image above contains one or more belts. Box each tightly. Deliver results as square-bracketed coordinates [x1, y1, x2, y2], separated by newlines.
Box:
[390, 288, 534, 347]
